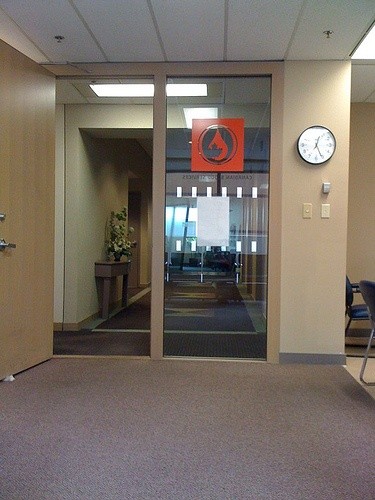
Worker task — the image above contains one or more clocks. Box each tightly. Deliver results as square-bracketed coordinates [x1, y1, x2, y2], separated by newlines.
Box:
[297, 126, 336, 165]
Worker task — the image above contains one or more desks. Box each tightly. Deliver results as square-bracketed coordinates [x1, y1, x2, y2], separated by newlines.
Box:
[95, 261, 131, 319]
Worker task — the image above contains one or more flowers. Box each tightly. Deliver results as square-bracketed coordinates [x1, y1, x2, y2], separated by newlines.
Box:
[105, 208, 137, 257]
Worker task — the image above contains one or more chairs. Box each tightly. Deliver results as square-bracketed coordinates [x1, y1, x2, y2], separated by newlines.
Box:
[358, 280, 375, 379]
[345, 276, 375, 358]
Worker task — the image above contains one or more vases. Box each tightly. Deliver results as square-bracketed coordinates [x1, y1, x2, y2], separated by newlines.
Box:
[114, 253, 122, 261]
